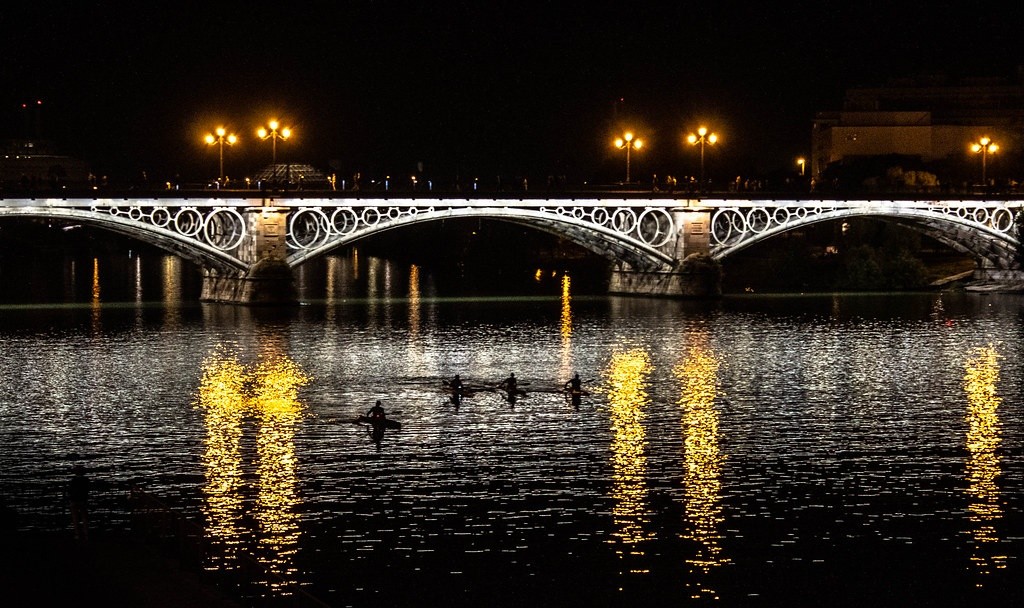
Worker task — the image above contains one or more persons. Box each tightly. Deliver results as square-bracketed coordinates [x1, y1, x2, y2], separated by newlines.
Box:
[450, 375, 463, 389]
[366, 400, 385, 419]
[499, 373, 517, 388]
[564, 374, 581, 391]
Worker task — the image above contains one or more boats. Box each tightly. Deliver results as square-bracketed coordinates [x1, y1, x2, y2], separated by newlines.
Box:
[445, 365, 590, 403]
[359, 413, 401, 428]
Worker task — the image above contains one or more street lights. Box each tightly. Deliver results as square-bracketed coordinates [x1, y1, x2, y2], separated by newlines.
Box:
[970, 136, 998, 184]
[688, 126, 717, 189]
[205, 127, 237, 189]
[615, 133, 643, 189]
[257, 118, 292, 189]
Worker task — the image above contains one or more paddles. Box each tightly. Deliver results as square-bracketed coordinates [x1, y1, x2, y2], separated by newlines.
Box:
[442, 380, 450, 386]
[368, 409, 404, 415]
[584, 379, 596, 384]
[518, 383, 530, 386]
